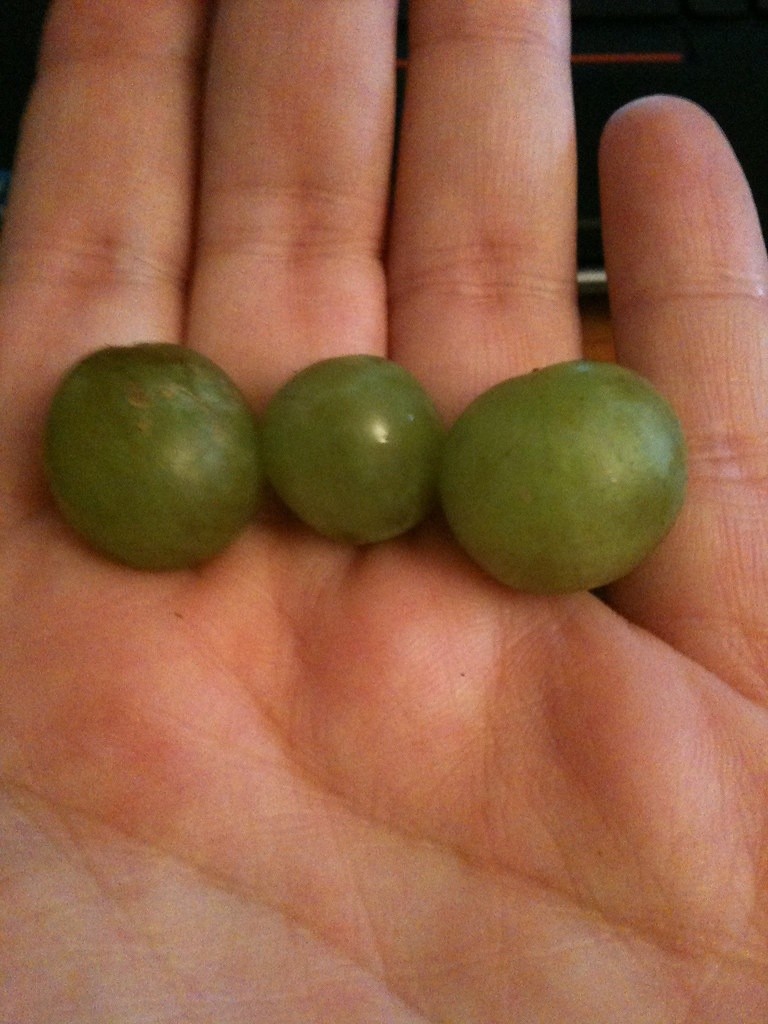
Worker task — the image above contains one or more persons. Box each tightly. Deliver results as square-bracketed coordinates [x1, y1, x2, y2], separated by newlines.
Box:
[0, 0, 768, 1024]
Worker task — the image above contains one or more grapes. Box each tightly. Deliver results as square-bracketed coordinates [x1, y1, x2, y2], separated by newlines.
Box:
[41, 342, 264, 569]
[436, 361, 687, 596]
[261, 352, 443, 546]
